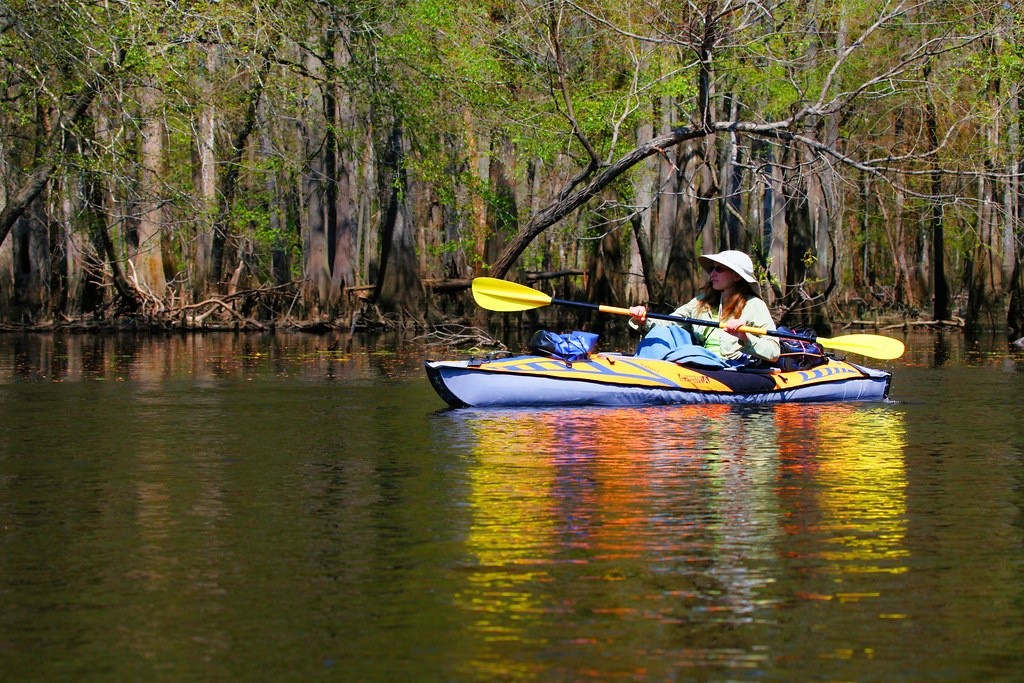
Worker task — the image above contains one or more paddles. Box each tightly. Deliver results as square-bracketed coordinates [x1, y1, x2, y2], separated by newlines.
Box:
[469, 276, 907, 363]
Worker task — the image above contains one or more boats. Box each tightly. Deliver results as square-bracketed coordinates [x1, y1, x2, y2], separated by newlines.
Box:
[424, 330, 893, 409]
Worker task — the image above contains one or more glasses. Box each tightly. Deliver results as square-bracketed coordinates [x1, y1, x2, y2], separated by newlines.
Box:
[709, 264, 732, 273]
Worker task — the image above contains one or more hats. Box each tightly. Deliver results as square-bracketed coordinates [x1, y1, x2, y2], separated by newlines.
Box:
[699, 250, 763, 299]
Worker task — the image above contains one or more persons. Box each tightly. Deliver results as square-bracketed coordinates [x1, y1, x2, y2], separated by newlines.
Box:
[628, 250, 780, 369]
